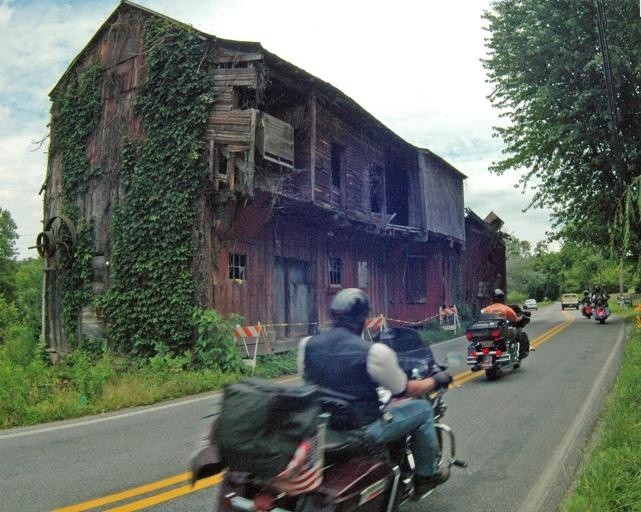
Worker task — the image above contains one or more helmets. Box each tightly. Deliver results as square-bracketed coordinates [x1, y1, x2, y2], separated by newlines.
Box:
[492, 288, 505, 302]
[328, 287, 372, 328]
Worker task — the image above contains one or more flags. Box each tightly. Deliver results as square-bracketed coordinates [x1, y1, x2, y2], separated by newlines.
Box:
[268, 436, 324, 497]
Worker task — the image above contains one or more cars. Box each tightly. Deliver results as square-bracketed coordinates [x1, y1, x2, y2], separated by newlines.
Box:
[525, 298, 538, 310]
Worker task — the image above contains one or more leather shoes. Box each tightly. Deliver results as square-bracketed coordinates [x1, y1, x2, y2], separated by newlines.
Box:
[414, 467, 451, 496]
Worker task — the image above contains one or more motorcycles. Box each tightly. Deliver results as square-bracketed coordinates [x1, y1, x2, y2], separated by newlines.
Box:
[216, 323, 471, 511]
[579, 296, 594, 319]
[592, 294, 613, 324]
[465, 301, 538, 381]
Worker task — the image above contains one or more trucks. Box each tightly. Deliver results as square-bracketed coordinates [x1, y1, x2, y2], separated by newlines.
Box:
[562, 292, 580, 310]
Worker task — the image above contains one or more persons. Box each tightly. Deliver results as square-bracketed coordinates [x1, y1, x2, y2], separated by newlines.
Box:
[580, 286, 608, 307]
[480, 289, 528, 358]
[297, 287, 452, 490]
